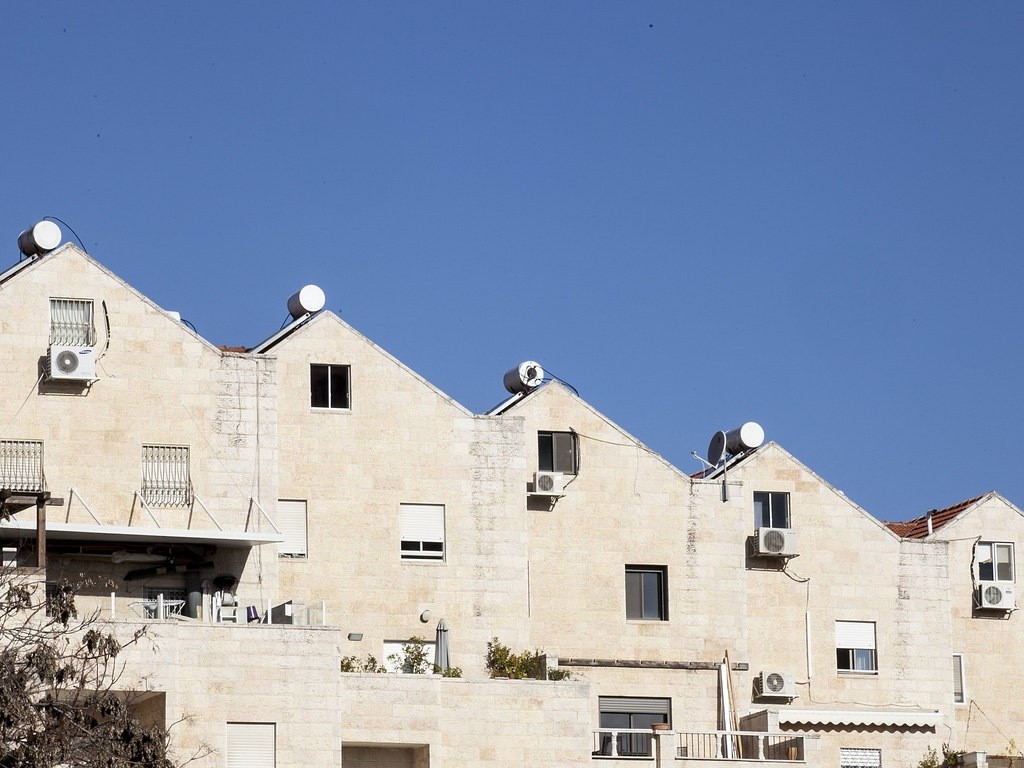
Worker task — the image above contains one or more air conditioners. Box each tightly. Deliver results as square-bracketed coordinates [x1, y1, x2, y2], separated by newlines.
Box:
[754, 526, 797, 557]
[533, 471, 563, 495]
[759, 671, 795, 696]
[47, 345, 96, 382]
[978, 581, 1016, 610]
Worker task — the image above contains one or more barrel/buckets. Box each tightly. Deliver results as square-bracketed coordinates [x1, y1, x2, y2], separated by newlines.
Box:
[246, 606, 260, 624]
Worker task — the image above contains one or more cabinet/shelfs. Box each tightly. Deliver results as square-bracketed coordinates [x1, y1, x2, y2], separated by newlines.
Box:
[218, 607, 238, 624]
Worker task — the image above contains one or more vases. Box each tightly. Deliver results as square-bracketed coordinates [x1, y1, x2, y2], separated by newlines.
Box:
[786, 746, 798, 760]
[650, 722, 670, 730]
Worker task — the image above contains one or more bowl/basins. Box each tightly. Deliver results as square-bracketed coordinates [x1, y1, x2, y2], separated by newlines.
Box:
[651, 723, 669, 730]
